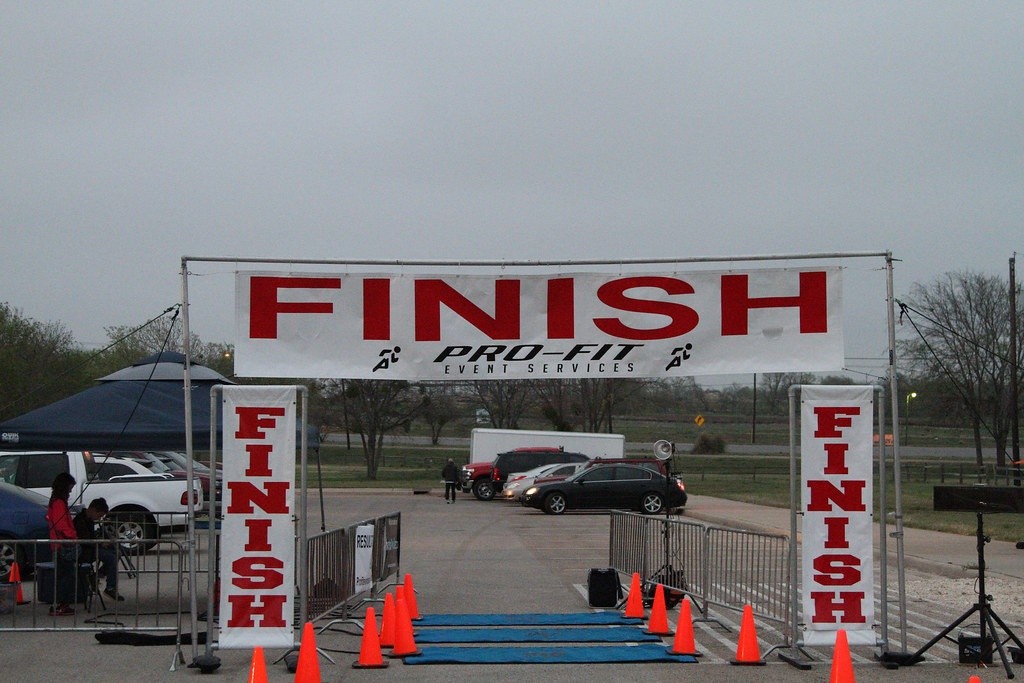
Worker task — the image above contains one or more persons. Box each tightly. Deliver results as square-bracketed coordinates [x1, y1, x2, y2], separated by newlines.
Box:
[74, 498, 124, 600]
[442, 458, 457, 504]
[48, 473, 77, 615]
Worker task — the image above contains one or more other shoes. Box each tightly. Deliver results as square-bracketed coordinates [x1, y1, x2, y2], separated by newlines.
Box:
[447, 499, 450, 504]
[50, 605, 75, 616]
[451, 500, 456, 504]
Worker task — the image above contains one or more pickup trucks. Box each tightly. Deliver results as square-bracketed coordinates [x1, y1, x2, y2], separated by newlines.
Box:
[0, 451, 205, 555]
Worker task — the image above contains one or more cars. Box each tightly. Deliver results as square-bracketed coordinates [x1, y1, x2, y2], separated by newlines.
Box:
[521, 463, 687, 515]
[501, 463, 585, 501]
[91, 451, 223, 517]
[0, 481, 87, 581]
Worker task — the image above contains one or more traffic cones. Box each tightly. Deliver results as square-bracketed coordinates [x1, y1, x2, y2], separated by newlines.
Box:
[404, 573, 424, 621]
[830, 629, 855, 683]
[293, 622, 321, 683]
[8, 562, 31, 605]
[968, 676, 980, 683]
[664, 598, 703, 656]
[379, 585, 424, 657]
[641, 584, 674, 636]
[621, 572, 649, 619]
[352, 607, 390, 669]
[729, 605, 766, 666]
[248, 646, 269, 683]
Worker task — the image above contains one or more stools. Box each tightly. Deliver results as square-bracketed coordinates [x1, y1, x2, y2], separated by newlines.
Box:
[34, 561, 90, 603]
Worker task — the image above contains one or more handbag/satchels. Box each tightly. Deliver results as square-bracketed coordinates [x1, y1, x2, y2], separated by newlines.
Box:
[60, 543, 82, 561]
[456, 482, 462, 491]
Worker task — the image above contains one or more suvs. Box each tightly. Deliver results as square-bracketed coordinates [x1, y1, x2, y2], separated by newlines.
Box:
[490, 451, 591, 492]
[461, 447, 562, 502]
[533, 456, 667, 484]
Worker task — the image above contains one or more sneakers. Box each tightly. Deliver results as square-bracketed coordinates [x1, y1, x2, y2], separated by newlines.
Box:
[86, 573, 101, 595]
[103, 588, 124, 601]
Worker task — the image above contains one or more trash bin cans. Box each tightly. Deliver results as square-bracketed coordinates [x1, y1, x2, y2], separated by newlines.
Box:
[0, 581, 21, 615]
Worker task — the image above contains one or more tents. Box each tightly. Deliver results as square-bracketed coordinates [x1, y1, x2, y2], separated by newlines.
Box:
[0, 350, 325, 541]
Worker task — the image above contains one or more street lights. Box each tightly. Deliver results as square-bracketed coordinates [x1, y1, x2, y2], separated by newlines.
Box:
[904, 393, 917, 445]
